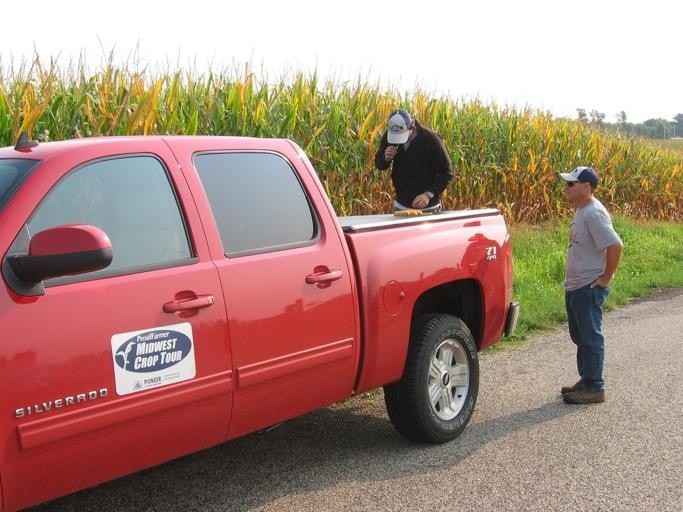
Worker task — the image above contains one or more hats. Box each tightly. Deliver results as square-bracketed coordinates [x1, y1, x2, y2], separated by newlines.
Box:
[386, 109, 413, 144]
[559, 166, 599, 188]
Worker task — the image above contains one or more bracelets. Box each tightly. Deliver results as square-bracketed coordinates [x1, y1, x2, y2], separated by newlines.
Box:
[424, 191, 434, 200]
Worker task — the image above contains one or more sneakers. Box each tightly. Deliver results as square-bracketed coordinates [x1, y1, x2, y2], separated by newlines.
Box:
[561, 380, 605, 403]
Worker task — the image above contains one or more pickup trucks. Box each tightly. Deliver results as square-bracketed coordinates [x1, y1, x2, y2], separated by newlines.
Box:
[0, 131, 520, 512]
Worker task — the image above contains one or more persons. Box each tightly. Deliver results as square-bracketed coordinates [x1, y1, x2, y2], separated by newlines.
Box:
[558, 165, 624, 404]
[373, 109, 454, 215]
